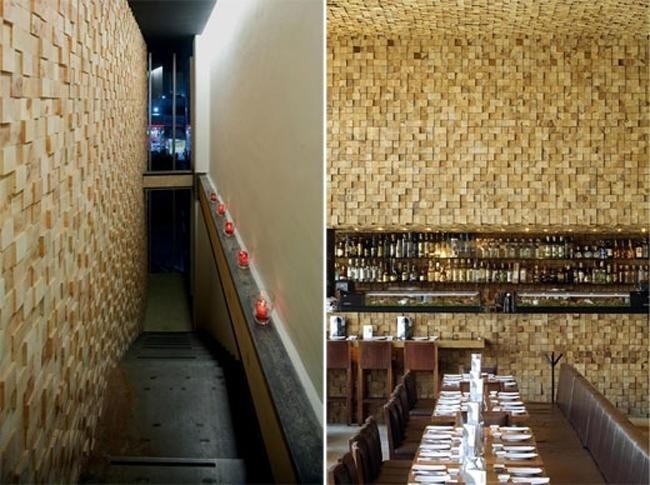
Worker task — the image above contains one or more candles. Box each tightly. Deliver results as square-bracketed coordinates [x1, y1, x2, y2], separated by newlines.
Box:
[210, 193, 268, 320]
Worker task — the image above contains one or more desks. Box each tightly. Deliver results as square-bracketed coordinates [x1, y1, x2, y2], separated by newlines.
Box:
[327, 336, 487, 398]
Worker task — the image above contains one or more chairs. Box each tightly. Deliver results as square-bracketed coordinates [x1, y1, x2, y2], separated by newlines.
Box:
[327, 340, 439, 426]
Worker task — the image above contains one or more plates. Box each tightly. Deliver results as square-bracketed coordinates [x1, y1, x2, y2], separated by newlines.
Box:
[413, 375, 549, 484]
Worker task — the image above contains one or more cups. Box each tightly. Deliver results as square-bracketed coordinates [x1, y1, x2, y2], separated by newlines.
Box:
[210, 192, 275, 326]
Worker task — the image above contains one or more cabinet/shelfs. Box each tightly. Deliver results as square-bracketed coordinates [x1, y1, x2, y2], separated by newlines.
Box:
[330, 230, 649, 314]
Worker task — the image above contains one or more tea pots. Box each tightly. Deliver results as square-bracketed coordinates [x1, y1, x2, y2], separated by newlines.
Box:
[396, 314, 413, 339]
[328, 315, 346, 340]
